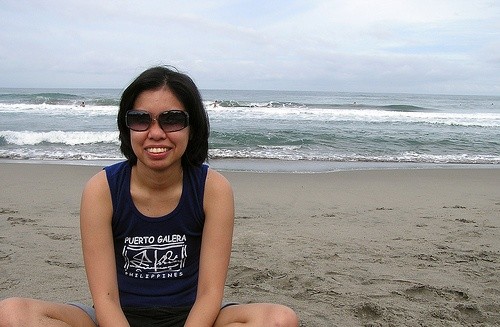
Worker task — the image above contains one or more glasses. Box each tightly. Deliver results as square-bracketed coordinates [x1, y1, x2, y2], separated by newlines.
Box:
[125, 109, 191, 132]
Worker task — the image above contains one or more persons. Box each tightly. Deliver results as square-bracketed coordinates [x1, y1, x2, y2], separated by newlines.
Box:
[0, 63, 301, 327]
